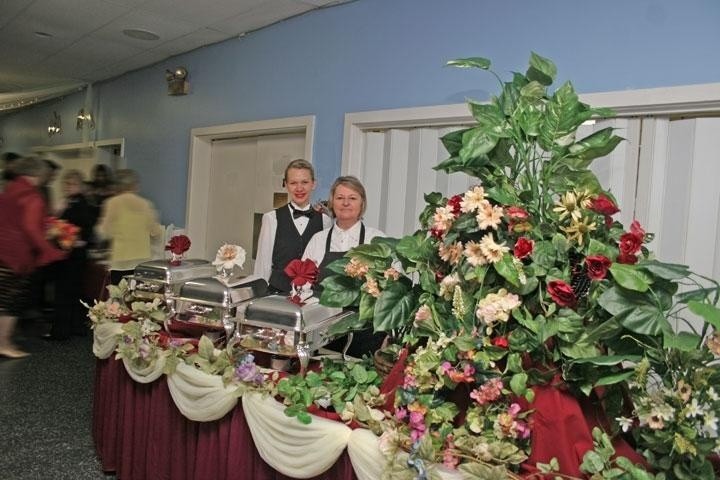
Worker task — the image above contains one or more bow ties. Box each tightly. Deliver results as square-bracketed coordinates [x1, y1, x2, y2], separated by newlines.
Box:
[292, 207, 315, 220]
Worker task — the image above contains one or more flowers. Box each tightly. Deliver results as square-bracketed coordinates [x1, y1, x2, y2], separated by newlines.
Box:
[77, 46, 719, 478]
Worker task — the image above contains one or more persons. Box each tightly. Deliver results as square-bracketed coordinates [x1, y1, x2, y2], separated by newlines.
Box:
[252, 157, 333, 299]
[1, 151, 24, 182]
[300, 174, 390, 359]
[35, 167, 91, 339]
[84, 164, 121, 230]
[34, 159, 62, 213]
[0, 155, 71, 359]
[93, 168, 164, 297]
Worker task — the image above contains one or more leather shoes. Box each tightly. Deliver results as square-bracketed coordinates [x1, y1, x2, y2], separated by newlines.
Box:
[0, 345, 33, 360]
